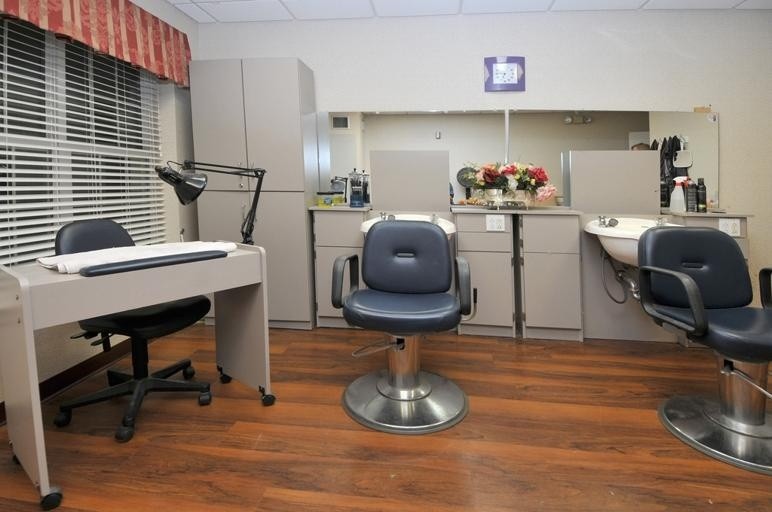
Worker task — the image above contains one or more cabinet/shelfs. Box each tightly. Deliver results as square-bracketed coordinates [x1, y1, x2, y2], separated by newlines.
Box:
[450, 208, 517, 338]
[662, 211, 754, 348]
[308, 205, 371, 329]
[517, 210, 583, 342]
[189, 57, 319, 330]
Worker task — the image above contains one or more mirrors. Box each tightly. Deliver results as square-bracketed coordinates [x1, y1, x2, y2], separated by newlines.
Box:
[329, 110, 505, 205]
[509, 111, 719, 210]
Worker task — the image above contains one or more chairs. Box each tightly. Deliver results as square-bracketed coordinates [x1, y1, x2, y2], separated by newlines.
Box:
[332, 221, 476, 435]
[55, 219, 211, 442]
[639, 226, 772, 475]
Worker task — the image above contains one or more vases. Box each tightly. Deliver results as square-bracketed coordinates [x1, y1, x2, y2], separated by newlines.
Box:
[484, 188, 502, 204]
[508, 189, 530, 209]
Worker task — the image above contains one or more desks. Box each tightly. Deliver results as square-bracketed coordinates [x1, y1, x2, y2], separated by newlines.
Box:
[0, 242, 275, 510]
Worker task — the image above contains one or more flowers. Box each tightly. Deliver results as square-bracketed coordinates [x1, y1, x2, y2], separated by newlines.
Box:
[465, 159, 510, 189]
[509, 158, 557, 201]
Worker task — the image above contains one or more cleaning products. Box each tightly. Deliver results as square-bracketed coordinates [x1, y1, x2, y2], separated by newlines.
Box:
[697, 178, 707, 212]
[670, 175, 689, 212]
[684, 178, 697, 212]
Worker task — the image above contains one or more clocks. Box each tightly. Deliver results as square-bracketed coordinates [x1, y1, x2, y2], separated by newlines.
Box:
[484, 57, 525, 92]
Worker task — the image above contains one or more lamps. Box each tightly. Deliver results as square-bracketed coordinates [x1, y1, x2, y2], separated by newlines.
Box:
[331, 176, 348, 202]
[155, 160, 266, 245]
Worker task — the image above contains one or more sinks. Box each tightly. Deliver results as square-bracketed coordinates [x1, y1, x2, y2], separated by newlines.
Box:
[360, 215, 456, 240]
[584, 218, 685, 267]
